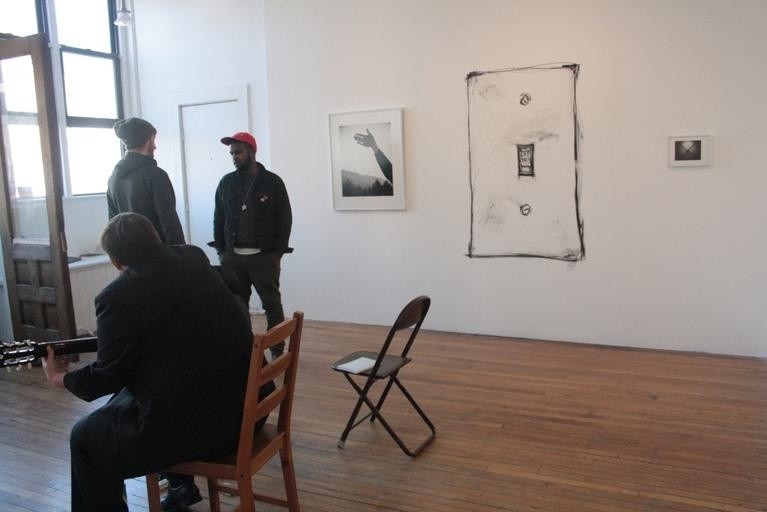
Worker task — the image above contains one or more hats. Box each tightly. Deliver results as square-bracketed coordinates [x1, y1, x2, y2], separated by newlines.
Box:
[113, 117, 157, 150]
[220, 132, 257, 152]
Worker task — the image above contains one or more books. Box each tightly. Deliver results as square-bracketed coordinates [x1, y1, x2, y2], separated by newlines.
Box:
[336, 355, 379, 375]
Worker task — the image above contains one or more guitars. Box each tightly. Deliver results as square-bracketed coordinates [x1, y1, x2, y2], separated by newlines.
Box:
[1, 334, 97, 372]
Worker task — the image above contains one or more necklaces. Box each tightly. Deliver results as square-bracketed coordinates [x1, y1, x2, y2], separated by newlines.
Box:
[237, 174, 257, 213]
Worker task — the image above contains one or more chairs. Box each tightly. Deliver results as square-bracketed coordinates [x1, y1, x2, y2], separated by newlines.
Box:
[145, 310, 304, 512]
[330, 294, 438, 458]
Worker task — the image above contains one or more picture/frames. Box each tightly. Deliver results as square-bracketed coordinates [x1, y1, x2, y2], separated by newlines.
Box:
[668, 136, 708, 167]
[328, 107, 406, 211]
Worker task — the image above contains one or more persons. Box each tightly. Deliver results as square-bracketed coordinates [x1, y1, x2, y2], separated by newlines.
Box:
[207, 132, 292, 361]
[106, 116, 187, 245]
[352, 128, 392, 186]
[39, 210, 279, 510]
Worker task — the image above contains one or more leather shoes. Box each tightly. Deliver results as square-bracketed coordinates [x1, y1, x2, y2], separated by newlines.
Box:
[159, 477, 203, 512]
